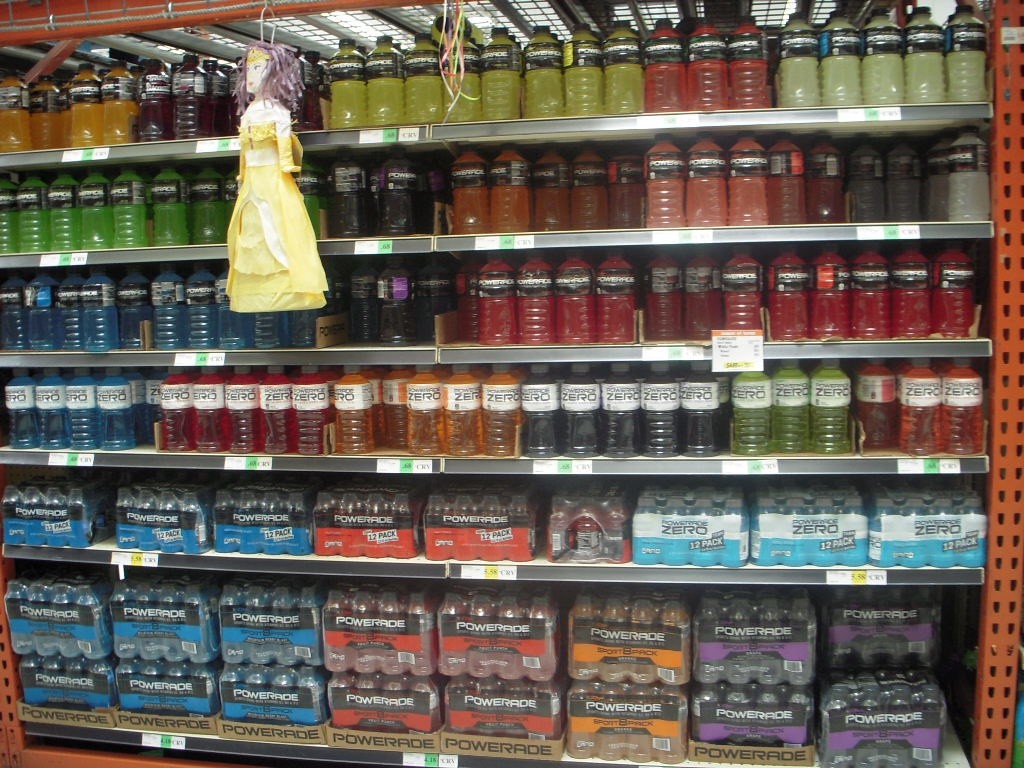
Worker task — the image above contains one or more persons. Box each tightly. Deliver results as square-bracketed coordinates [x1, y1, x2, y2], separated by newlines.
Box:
[225, 40, 329, 314]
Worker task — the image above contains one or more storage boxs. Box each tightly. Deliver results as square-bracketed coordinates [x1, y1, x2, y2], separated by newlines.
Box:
[18, 697, 566, 760]
[686, 731, 817, 764]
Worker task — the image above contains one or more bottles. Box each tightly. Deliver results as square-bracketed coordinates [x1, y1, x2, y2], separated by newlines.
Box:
[0, 4, 991, 768]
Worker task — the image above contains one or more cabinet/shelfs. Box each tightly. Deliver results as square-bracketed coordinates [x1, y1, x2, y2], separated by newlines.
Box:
[0, 0, 1024, 767]
